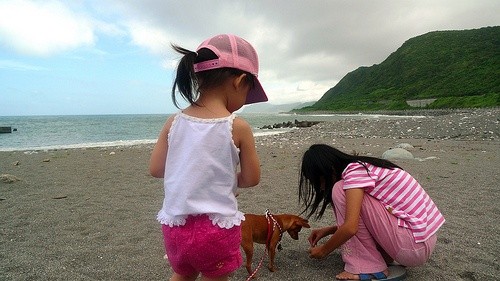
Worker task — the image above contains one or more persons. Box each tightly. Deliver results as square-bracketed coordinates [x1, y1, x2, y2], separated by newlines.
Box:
[149, 33, 269, 281]
[296, 143, 447, 281]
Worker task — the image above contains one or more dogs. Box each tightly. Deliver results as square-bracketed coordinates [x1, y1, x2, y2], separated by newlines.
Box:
[241, 214, 311, 279]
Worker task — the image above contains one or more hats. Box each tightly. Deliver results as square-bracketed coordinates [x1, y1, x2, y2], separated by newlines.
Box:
[193, 34, 268, 104]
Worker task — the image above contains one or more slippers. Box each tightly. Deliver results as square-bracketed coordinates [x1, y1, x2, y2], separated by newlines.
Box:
[358, 266, 407, 281]
[386, 260, 405, 266]
